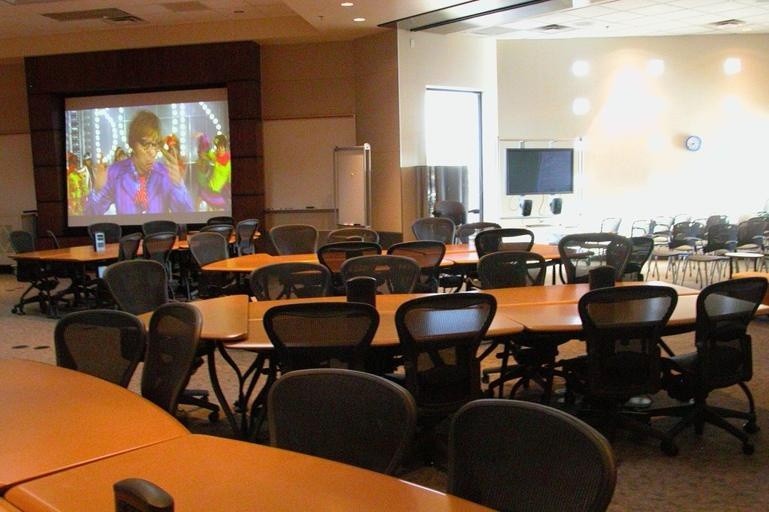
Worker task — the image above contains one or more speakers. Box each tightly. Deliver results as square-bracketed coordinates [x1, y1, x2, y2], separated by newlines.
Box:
[523, 200, 532, 216]
[552, 198, 561, 214]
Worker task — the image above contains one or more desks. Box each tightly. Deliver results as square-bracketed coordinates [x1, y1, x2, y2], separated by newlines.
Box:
[0, 434, 489, 512]
[726, 252, 764, 279]
[135, 280, 762, 442]
[1, 352, 193, 492]
[203, 242, 593, 296]
[20, 229, 263, 312]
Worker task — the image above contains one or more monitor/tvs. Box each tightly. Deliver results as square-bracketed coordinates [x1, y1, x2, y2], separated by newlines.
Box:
[506, 148, 573, 195]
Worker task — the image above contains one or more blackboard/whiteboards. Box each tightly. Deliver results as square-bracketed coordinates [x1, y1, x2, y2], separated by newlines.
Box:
[333, 143, 372, 227]
[263, 114, 356, 212]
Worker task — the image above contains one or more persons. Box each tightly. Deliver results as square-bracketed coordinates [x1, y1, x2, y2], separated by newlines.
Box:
[113, 145, 128, 162]
[80, 150, 97, 193]
[80, 109, 195, 216]
[65, 153, 88, 216]
[199, 133, 230, 211]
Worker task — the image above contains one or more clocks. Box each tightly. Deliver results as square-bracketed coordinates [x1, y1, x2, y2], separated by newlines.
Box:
[685, 136, 701, 151]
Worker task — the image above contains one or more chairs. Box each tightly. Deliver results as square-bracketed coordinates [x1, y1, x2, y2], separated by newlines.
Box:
[88, 221, 121, 244]
[413, 215, 463, 276]
[261, 303, 381, 364]
[623, 236, 654, 280]
[234, 218, 260, 257]
[476, 228, 536, 258]
[10, 228, 73, 315]
[386, 239, 447, 293]
[645, 221, 701, 284]
[574, 217, 622, 270]
[150, 301, 222, 425]
[318, 241, 383, 273]
[140, 322, 197, 417]
[681, 222, 738, 289]
[199, 223, 234, 242]
[340, 255, 419, 294]
[394, 294, 496, 399]
[139, 219, 179, 236]
[664, 276, 764, 456]
[455, 223, 505, 241]
[187, 230, 230, 265]
[270, 222, 319, 299]
[143, 230, 179, 262]
[207, 215, 237, 226]
[560, 285, 676, 399]
[737, 219, 767, 251]
[266, 368, 413, 476]
[433, 201, 476, 237]
[447, 400, 619, 510]
[47, 225, 82, 279]
[53, 311, 145, 387]
[559, 231, 635, 280]
[325, 227, 380, 245]
[247, 262, 333, 301]
[118, 232, 144, 257]
[99, 258, 171, 312]
[465, 251, 547, 291]
